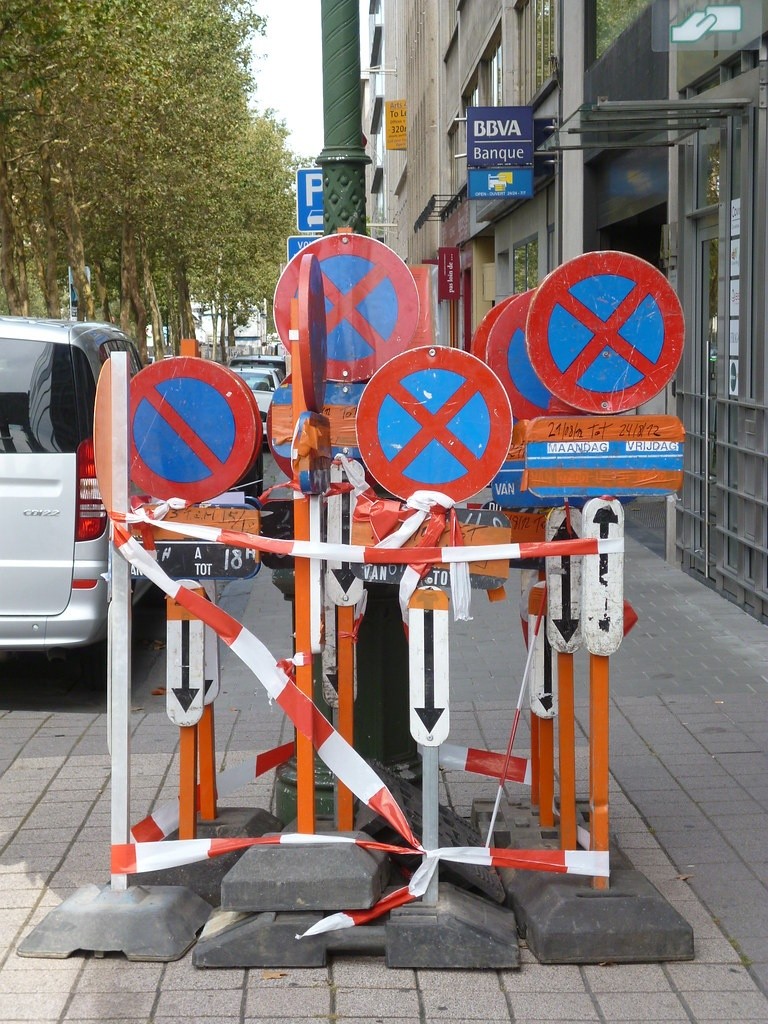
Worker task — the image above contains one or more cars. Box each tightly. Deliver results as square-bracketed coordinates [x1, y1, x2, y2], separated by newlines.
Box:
[0, 311, 152, 659]
[226, 353, 285, 445]
